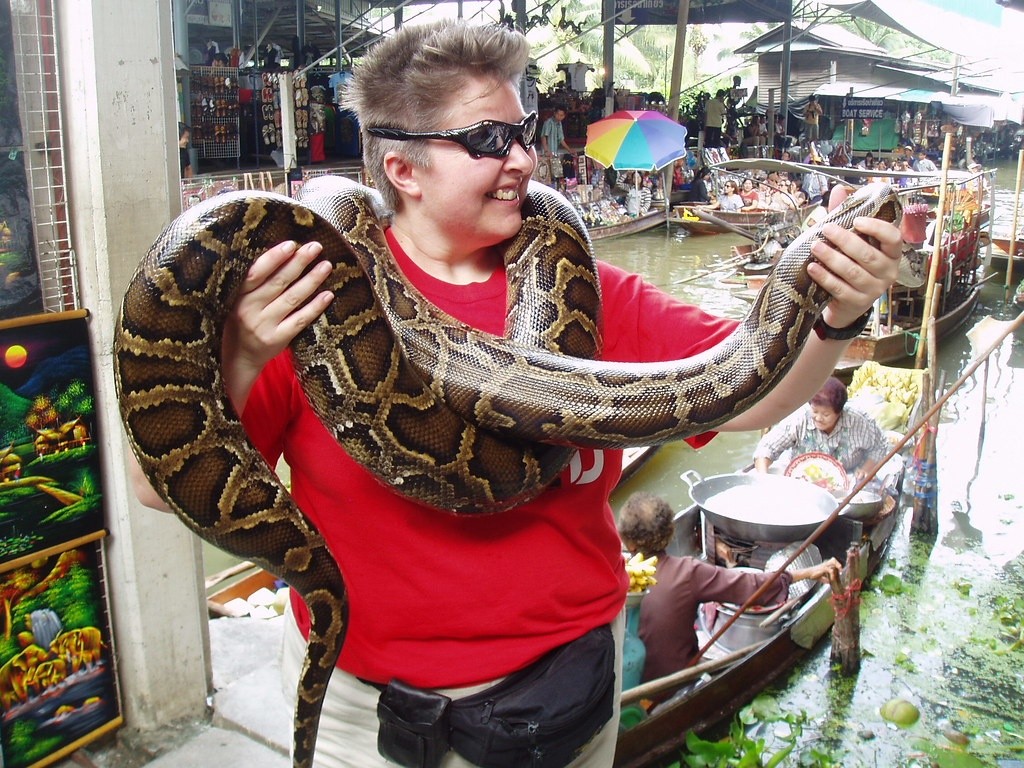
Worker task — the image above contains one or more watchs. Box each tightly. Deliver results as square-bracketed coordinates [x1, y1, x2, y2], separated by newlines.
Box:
[813, 304, 874, 340]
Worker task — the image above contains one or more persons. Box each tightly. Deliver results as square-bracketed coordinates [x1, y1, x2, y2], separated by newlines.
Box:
[138, 21, 904, 767]
[616, 490, 843, 704]
[753, 377, 887, 488]
[178, 122, 192, 179]
[539, 89, 1024, 215]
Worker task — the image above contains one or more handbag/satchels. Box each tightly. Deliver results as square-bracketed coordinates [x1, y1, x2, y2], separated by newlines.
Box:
[451, 623, 615, 768]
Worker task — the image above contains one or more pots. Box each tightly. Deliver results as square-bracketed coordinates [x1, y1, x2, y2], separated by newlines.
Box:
[680, 470, 852, 545]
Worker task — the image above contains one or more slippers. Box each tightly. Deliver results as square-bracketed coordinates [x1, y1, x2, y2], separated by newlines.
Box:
[262, 70, 308, 147]
[190, 75, 239, 144]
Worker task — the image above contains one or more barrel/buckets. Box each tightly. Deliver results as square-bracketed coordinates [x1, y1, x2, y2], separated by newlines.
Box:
[699, 602, 780, 657]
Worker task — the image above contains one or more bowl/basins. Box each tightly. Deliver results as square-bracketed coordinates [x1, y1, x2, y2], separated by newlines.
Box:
[844, 490, 881, 519]
[624, 589, 650, 605]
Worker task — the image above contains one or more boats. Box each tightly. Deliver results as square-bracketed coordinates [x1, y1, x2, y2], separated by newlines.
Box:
[830, 156, 995, 378]
[986, 224, 1024, 270]
[669, 158, 827, 236]
[887, 163, 1000, 233]
[613, 430, 907, 768]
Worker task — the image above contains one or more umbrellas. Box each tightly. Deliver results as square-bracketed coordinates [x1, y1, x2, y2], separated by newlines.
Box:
[585, 110, 687, 217]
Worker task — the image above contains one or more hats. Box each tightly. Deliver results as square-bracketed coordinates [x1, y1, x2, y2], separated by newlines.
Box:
[769, 174, 778, 182]
[904, 145, 913, 151]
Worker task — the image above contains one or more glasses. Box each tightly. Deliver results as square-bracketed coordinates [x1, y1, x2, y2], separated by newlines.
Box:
[725, 185, 731, 188]
[365, 111, 539, 159]
